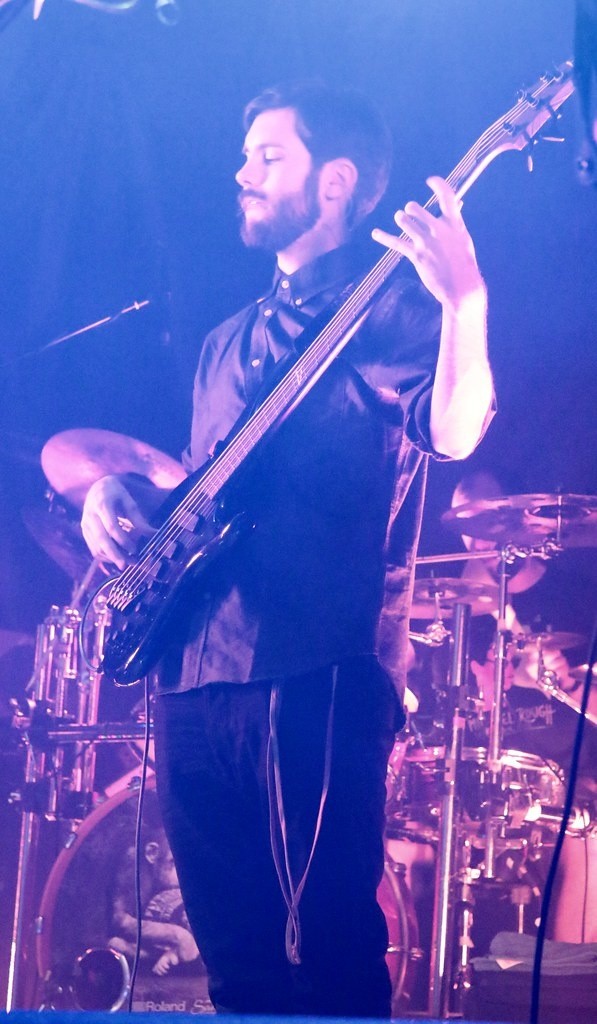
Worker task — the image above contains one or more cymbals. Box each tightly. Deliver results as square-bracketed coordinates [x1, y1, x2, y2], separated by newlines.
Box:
[439, 489, 596, 551]
[39, 429, 193, 514]
[409, 577, 511, 622]
[506, 630, 589, 650]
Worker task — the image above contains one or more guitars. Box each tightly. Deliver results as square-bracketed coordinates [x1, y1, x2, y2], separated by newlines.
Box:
[79, 59, 585, 685]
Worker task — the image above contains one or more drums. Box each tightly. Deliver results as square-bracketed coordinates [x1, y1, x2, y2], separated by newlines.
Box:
[35, 771, 422, 1021]
[396, 744, 566, 849]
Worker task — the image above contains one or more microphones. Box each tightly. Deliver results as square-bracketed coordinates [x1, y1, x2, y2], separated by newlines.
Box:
[101, 290, 172, 328]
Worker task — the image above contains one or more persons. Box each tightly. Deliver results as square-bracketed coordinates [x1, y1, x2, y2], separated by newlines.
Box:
[383, 465, 597, 1013]
[81, 85, 496, 1023]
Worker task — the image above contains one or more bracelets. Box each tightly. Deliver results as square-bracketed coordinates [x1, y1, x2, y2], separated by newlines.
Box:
[561, 679, 582, 696]
